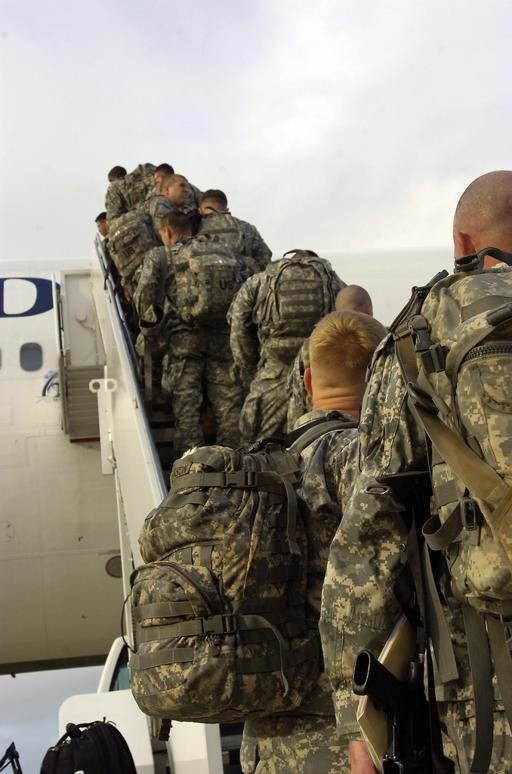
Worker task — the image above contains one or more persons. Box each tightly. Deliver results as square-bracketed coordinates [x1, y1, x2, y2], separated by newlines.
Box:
[317, 168, 510, 773]
[95, 159, 374, 454]
[232, 308, 389, 774]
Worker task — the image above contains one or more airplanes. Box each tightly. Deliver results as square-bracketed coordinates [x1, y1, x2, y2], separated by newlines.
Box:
[0, 245, 491, 678]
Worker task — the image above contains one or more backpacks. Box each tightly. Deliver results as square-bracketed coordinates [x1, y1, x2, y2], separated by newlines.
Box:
[125, 163, 156, 205]
[175, 239, 242, 322]
[127, 446, 321, 726]
[416, 243, 512, 615]
[258, 248, 330, 338]
[105, 209, 157, 281]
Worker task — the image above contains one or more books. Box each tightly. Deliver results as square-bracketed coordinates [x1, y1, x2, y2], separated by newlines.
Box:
[358, 614, 414, 772]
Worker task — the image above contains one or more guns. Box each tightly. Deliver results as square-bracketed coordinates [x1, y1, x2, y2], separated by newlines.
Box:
[351, 653, 456, 772]
